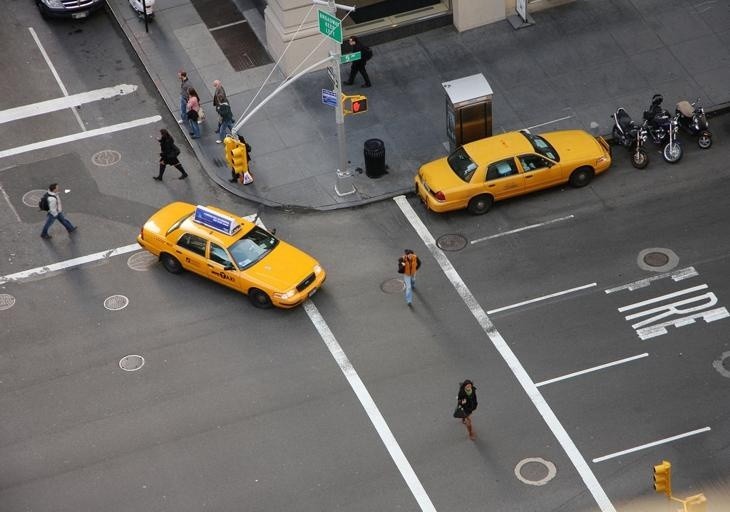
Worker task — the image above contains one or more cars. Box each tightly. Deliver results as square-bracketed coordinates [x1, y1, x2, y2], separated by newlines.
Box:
[409, 126, 613, 218]
[38, 0, 105, 19]
[135, 199, 329, 312]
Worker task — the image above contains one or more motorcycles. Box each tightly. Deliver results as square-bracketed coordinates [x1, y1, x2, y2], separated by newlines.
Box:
[672, 95, 714, 151]
[127, 0, 156, 24]
[640, 91, 685, 164]
[608, 102, 650, 170]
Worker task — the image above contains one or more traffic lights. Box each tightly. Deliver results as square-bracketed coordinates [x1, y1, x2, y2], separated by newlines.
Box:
[350, 95, 370, 116]
[222, 136, 232, 171]
[231, 140, 248, 176]
[651, 458, 672, 494]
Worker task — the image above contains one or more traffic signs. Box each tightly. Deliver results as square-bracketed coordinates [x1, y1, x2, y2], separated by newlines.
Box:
[317, 10, 345, 45]
[320, 88, 337, 107]
[326, 49, 337, 65]
[340, 51, 363, 64]
[325, 66, 335, 83]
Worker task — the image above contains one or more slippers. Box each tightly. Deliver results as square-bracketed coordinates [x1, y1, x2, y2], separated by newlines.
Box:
[175, 164, 188, 179]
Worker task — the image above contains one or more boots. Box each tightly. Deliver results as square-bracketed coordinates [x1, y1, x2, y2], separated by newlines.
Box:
[153, 164, 165, 180]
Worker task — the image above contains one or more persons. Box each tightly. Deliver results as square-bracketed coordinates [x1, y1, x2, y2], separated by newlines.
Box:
[184, 87, 202, 140]
[176, 69, 195, 125]
[341, 36, 373, 89]
[215, 94, 235, 144]
[523, 158, 536, 172]
[152, 128, 190, 182]
[39, 183, 80, 241]
[225, 134, 253, 184]
[398, 249, 422, 307]
[211, 79, 234, 133]
[453, 378, 480, 438]
[646, 95, 673, 130]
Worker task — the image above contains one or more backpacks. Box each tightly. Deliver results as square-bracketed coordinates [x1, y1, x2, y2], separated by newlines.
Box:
[39, 192, 57, 212]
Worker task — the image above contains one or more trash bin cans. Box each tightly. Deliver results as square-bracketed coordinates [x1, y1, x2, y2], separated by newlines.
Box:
[364, 138, 385, 180]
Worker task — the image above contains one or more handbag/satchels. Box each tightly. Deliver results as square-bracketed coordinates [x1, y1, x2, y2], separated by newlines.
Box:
[398, 256, 404, 273]
[453, 403, 467, 418]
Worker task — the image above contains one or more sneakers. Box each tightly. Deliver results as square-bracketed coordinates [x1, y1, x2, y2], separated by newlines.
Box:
[43, 236, 51, 239]
[70, 226, 77, 232]
[216, 140, 223, 144]
[178, 120, 184, 124]
